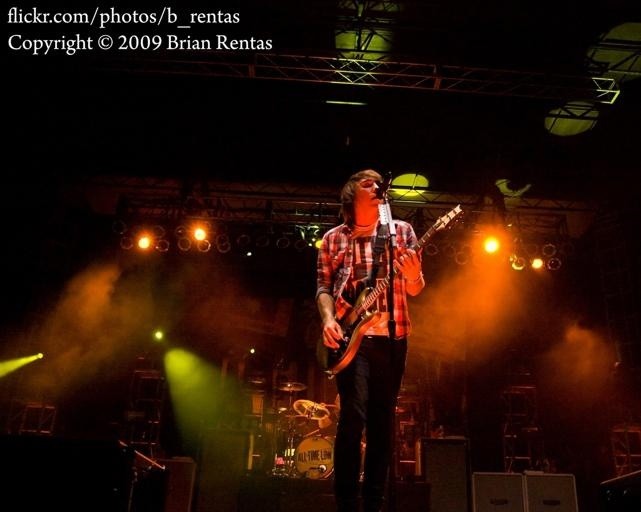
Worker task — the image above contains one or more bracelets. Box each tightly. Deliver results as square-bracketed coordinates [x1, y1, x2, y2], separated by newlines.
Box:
[404, 273, 422, 285]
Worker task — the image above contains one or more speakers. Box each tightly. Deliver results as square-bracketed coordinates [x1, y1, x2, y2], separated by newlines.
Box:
[194, 429, 253, 512]
[416, 437, 469, 511]
[598, 469, 641, 512]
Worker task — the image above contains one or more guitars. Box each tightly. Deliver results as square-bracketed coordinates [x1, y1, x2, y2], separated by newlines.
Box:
[316, 201, 464, 375]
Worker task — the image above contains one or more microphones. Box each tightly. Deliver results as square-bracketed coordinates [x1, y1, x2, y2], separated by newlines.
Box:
[376, 172, 391, 198]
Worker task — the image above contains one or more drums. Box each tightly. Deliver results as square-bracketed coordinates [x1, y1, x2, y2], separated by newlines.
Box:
[291, 400, 331, 434]
[294, 434, 336, 479]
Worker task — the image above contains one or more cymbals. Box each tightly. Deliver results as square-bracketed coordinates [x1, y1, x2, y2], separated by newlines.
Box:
[279, 382, 303, 391]
[297, 402, 324, 418]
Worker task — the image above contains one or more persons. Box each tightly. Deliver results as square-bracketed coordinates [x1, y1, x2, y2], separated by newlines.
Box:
[315, 168, 427, 512]
